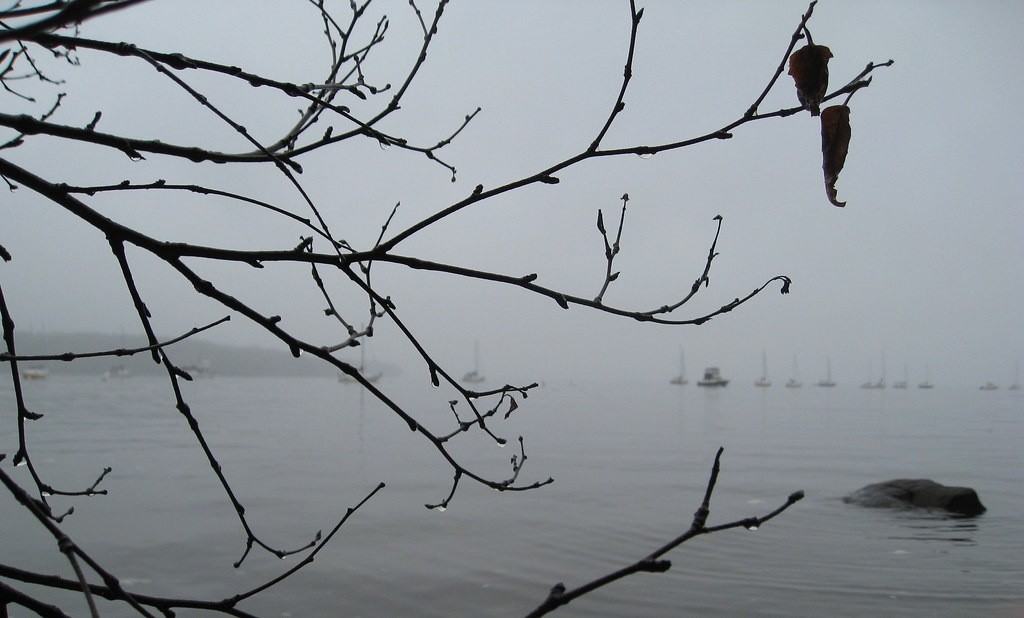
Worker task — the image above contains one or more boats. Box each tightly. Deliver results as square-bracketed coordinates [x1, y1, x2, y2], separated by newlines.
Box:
[694, 364, 731, 388]
